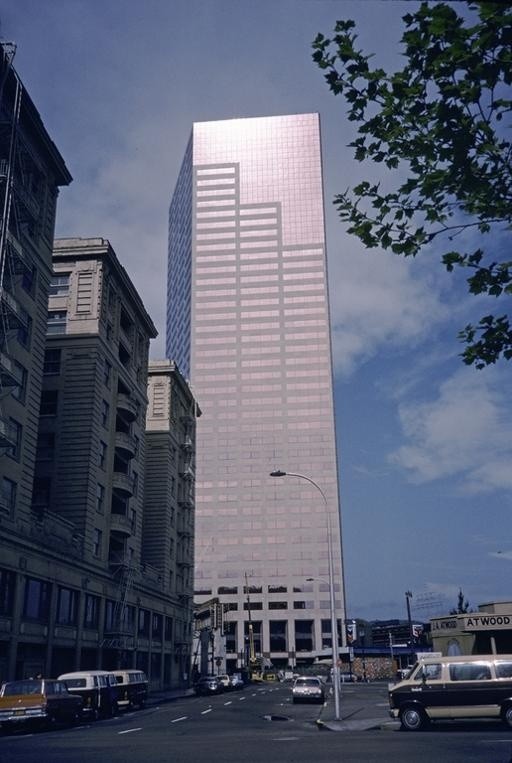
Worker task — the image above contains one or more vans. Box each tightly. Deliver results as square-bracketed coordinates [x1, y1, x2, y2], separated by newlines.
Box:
[1, 667, 153, 736]
[387, 653, 512, 733]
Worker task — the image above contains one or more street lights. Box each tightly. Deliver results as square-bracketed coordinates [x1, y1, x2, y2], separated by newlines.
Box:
[304, 575, 344, 699]
[268, 469, 346, 722]
[404, 586, 418, 666]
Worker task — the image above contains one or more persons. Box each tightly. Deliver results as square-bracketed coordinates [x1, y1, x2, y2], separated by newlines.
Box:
[426, 664, 440, 679]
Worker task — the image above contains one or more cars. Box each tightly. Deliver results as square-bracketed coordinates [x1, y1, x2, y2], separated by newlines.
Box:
[330, 672, 364, 683]
[290, 676, 326, 706]
[193, 674, 246, 698]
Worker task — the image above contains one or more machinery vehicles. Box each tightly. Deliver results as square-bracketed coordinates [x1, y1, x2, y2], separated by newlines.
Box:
[243, 571, 279, 685]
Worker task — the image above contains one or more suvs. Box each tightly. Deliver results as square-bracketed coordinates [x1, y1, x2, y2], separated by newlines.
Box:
[396, 664, 416, 681]
